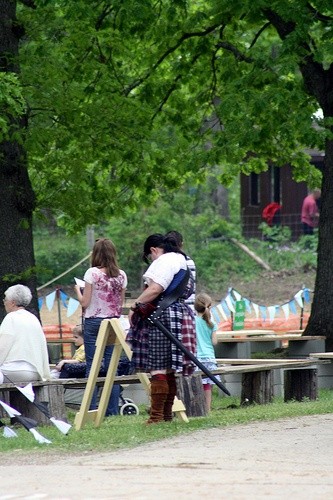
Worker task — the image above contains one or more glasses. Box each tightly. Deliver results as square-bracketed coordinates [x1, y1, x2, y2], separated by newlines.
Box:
[2, 299, 9, 303]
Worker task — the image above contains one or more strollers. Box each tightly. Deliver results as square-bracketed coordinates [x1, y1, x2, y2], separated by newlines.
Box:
[56, 350, 139, 418]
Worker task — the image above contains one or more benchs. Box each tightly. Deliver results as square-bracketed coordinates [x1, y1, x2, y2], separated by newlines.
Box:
[0, 329, 333, 424]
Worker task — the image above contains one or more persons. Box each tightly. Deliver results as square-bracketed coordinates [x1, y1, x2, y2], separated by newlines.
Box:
[164, 230, 197, 314]
[0, 284, 51, 383]
[191, 293, 222, 411]
[50, 323, 87, 378]
[74, 238, 128, 417]
[301, 187, 321, 235]
[127, 233, 197, 425]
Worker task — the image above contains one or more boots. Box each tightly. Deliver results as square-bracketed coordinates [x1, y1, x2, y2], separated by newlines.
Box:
[144, 379, 169, 425]
[162, 380, 176, 423]
